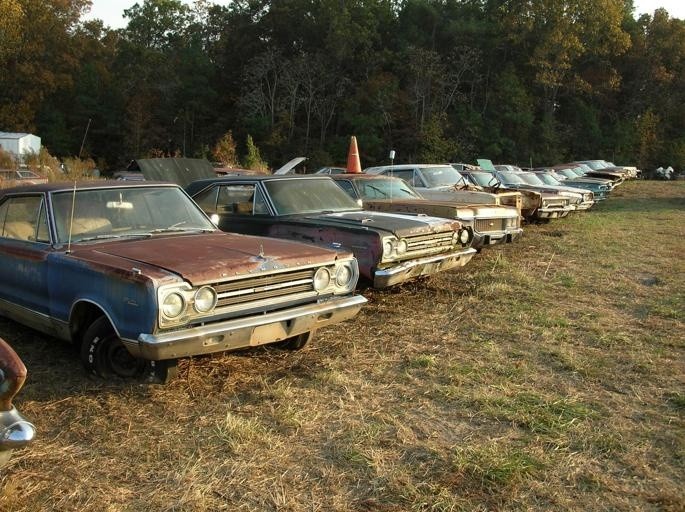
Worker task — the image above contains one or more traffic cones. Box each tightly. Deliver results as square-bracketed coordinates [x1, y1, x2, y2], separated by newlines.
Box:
[344, 134, 366, 177]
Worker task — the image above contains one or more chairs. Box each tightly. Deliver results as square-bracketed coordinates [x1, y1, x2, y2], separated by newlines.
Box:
[0, 217, 113, 242]
[235, 201, 261, 214]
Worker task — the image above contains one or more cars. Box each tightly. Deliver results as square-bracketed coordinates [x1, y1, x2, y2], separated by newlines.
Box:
[0, 170, 49, 186]
[111, 171, 146, 182]
[135, 159, 478, 289]
[324, 171, 523, 253]
[214, 160, 646, 222]
[0, 179, 369, 385]
